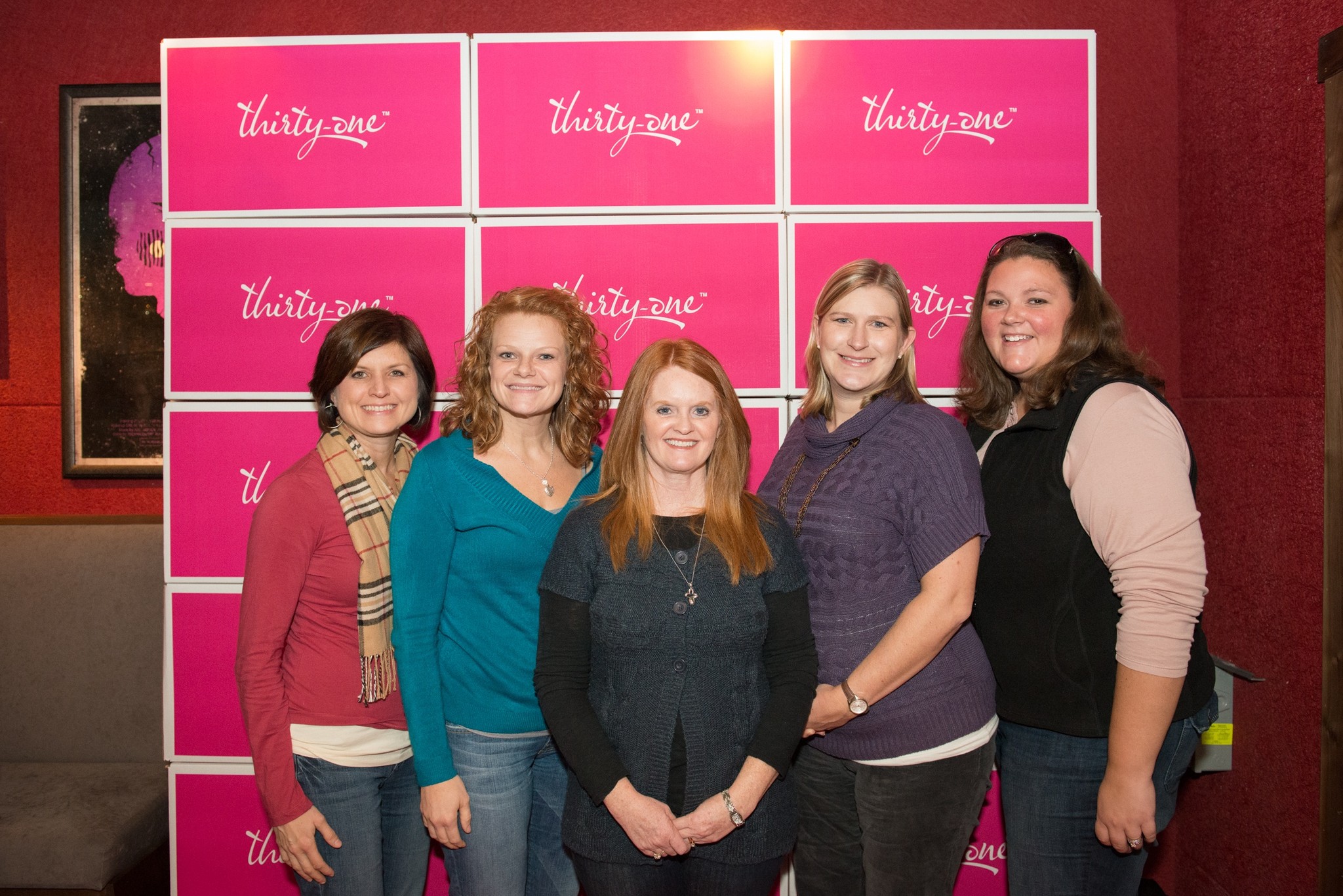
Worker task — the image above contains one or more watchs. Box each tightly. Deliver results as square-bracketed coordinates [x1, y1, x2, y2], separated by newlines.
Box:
[721, 788, 745, 827]
[841, 679, 869, 715]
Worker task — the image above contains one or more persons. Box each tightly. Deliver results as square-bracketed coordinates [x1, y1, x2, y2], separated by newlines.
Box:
[389, 287, 612, 896]
[533, 337, 819, 896]
[756, 260, 990, 895]
[235, 309, 434, 896]
[957, 232, 1218, 896]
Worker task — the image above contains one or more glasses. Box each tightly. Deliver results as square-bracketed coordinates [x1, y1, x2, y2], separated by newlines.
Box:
[987, 231, 1077, 271]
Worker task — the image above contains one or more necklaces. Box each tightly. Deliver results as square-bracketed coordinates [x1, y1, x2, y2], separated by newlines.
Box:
[650, 512, 706, 605]
[500, 426, 554, 497]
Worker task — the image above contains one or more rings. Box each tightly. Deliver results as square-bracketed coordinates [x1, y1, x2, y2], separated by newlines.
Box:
[688, 837, 695, 847]
[1127, 837, 1144, 848]
[653, 851, 664, 859]
